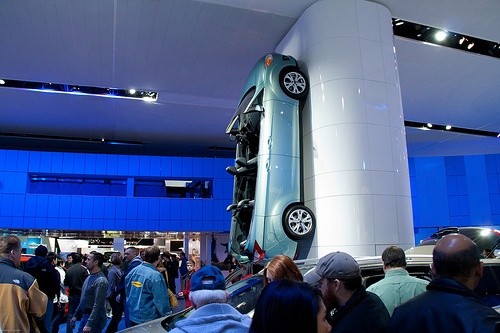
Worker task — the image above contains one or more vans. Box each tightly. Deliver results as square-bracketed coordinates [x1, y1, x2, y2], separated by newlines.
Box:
[115, 227, 500, 333]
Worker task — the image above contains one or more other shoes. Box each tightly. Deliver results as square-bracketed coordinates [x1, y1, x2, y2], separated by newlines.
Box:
[106, 313, 113, 319]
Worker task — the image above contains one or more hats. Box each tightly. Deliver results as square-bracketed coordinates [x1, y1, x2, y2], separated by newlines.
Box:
[190, 264, 227, 292]
[304, 251, 361, 284]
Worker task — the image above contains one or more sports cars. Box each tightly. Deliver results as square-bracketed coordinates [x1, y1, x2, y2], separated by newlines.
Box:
[225, 51, 315, 268]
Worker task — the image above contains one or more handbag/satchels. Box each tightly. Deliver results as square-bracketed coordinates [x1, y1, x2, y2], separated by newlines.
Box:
[167, 289, 178, 309]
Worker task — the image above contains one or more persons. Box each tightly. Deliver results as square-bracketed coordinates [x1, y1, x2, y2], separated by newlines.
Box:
[0, 232, 500, 333]
